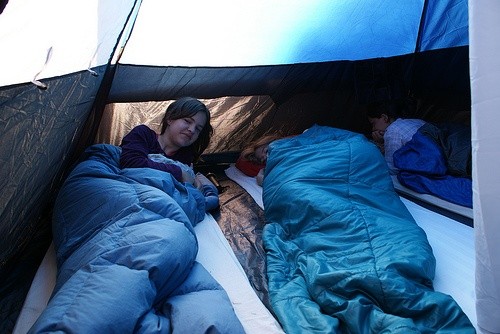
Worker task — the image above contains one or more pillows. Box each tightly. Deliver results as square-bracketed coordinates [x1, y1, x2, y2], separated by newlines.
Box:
[236, 134, 299, 177]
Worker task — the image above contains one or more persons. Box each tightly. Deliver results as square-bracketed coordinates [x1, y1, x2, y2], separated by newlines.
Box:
[117, 96, 213, 185]
[366, 99, 398, 152]
[237, 124, 304, 188]
